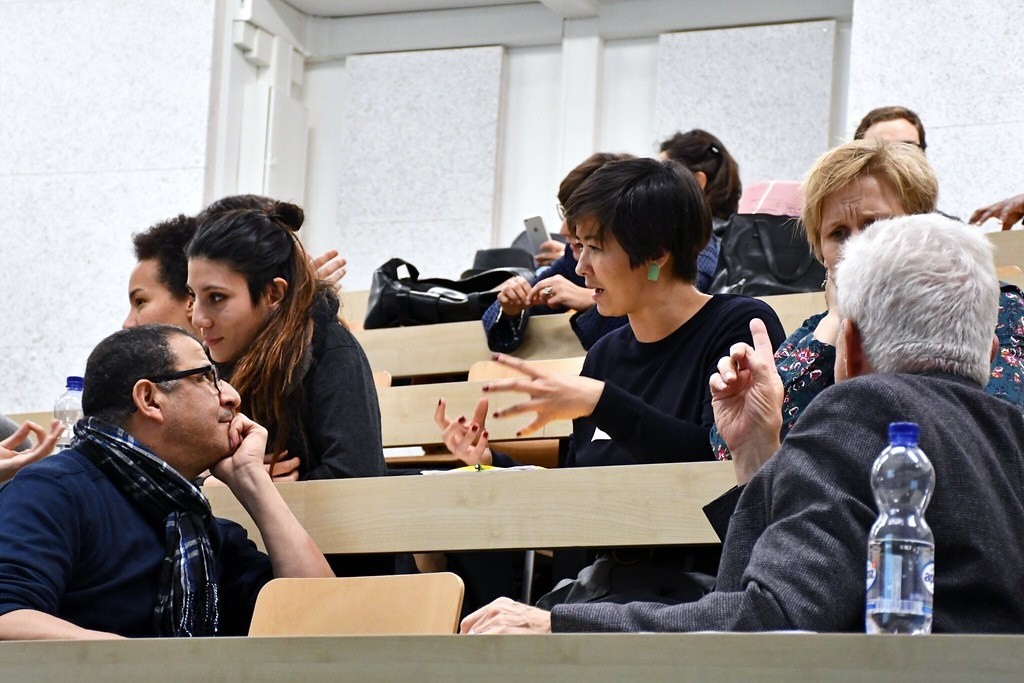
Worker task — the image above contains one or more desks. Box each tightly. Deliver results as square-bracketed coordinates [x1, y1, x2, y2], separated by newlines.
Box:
[178, 291, 832, 615]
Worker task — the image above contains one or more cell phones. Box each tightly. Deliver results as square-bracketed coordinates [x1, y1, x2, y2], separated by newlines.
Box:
[523, 214, 552, 256]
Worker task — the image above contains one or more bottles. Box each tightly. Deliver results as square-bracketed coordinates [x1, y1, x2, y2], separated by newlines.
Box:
[54, 376, 83, 454]
[866, 422, 937, 637]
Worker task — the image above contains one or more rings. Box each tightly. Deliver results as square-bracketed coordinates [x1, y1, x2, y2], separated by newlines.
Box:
[552, 256, 556, 259]
[548, 261, 552, 265]
[57, 436, 60, 440]
[468, 629, 474, 634]
[542, 287, 552, 299]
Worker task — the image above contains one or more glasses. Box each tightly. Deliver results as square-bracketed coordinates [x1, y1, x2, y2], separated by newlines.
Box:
[131, 364, 221, 412]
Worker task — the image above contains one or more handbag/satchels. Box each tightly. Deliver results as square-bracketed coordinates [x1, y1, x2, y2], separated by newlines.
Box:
[710, 213, 827, 296]
[364, 258, 535, 330]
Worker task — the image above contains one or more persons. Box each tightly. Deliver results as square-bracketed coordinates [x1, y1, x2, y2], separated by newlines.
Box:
[458, 211, 1023, 635]
[0, 320, 337, 641]
[182, 192, 421, 579]
[433, 152, 786, 605]
[121, 215, 208, 373]
[709, 140, 1024, 460]
[822, 104, 1024, 292]
[662, 128, 825, 298]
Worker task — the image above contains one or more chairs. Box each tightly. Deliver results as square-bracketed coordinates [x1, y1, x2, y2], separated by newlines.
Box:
[246, 349, 587, 638]
